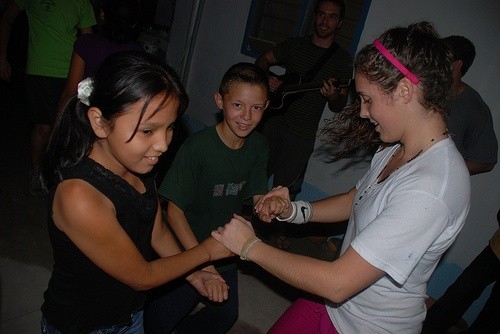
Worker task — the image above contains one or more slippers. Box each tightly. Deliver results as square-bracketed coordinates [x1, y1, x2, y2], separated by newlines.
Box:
[276, 234, 290, 250]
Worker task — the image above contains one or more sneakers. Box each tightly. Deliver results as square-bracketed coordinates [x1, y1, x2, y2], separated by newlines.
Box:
[29, 182, 39, 196]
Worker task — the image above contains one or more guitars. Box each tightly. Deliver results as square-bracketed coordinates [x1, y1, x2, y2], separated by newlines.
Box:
[255, 68, 357, 112]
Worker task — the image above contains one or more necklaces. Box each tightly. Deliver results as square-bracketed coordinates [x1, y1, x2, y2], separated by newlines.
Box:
[372, 128, 449, 185]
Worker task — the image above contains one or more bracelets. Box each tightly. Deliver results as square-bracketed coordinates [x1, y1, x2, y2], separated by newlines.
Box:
[276, 200, 313, 225]
[240, 236, 262, 260]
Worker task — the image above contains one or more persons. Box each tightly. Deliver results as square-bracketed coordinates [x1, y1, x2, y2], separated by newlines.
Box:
[143, 62, 290, 334]
[439, 35, 498, 176]
[34, 51, 239, 334]
[254, 0, 354, 249]
[56, 33, 123, 116]
[0, 0, 97, 197]
[211, 21, 472, 334]
